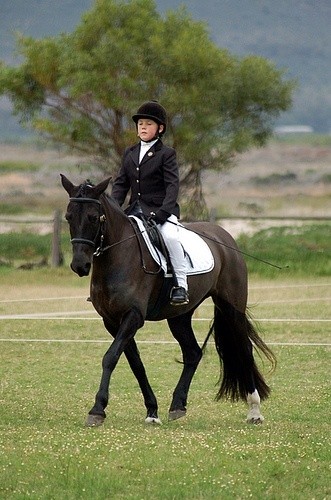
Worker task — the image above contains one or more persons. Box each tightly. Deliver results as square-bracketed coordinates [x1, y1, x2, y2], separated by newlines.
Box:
[87, 98, 189, 306]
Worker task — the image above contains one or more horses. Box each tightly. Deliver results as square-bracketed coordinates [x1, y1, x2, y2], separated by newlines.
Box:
[60, 174, 279, 429]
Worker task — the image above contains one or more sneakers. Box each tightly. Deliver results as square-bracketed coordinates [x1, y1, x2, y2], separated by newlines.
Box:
[172, 288, 187, 302]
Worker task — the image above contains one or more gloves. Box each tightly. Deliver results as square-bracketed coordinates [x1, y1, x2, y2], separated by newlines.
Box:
[152, 210, 170, 225]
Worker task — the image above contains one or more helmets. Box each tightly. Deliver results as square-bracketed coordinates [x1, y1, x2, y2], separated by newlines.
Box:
[131, 102, 167, 126]
